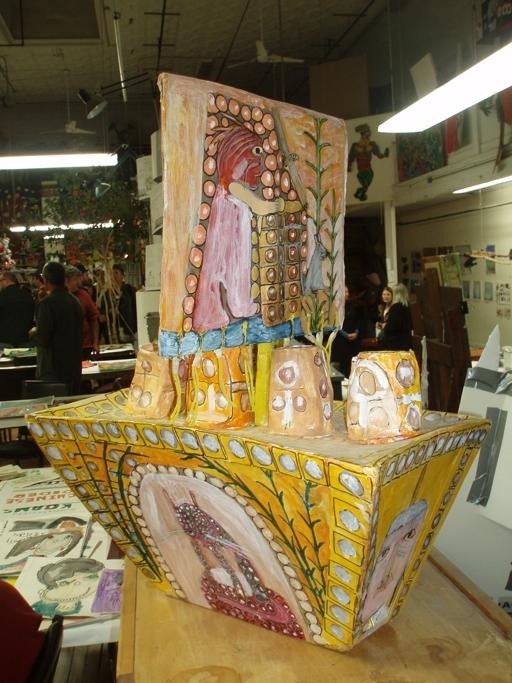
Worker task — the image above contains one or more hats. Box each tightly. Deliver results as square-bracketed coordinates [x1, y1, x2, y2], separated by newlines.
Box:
[65, 265, 82, 275]
[74, 262, 88, 272]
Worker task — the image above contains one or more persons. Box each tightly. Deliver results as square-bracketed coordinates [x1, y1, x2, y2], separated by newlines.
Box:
[0, 259, 136, 394]
[375, 287, 393, 341]
[337, 281, 363, 376]
[347, 122, 390, 201]
[381, 282, 415, 351]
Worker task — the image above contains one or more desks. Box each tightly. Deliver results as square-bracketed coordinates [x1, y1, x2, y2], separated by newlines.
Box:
[0, 343, 137, 468]
[116, 545, 512, 683]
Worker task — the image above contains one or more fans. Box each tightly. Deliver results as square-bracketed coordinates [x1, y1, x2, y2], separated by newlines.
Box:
[228, 40, 304, 68]
[40, 120, 96, 135]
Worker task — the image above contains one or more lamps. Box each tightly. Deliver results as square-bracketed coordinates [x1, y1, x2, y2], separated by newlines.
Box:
[0, 60, 159, 232]
[377, 0, 512, 133]
